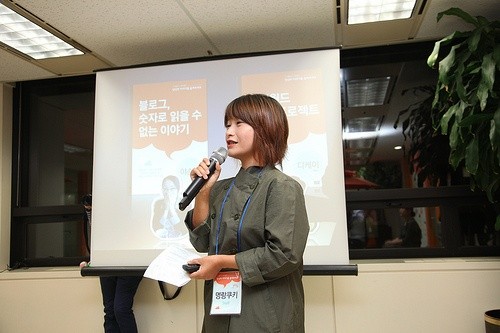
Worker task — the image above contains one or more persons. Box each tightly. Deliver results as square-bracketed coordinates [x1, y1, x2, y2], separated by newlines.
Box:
[384, 206, 423, 248]
[80, 203, 144, 333]
[152, 176, 188, 238]
[184, 93, 310, 333]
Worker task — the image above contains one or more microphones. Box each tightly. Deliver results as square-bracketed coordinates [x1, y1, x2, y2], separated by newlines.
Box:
[179, 147, 227, 211]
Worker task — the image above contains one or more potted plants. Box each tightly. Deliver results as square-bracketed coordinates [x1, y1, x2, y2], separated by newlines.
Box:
[427, 7, 500, 333]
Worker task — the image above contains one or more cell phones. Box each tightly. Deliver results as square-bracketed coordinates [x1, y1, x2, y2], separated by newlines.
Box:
[183, 264, 200, 273]
